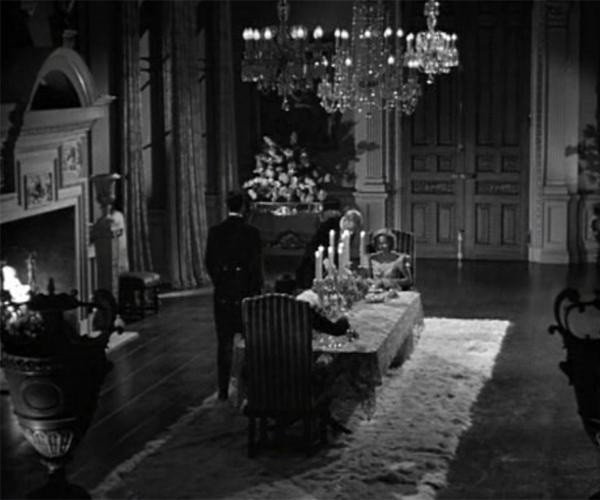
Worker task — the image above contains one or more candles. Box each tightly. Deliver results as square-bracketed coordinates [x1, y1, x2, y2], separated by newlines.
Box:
[309, 226, 365, 282]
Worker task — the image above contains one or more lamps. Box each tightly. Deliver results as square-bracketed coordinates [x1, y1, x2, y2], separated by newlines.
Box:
[316, 1, 423, 123]
[236, 1, 328, 113]
[404, 1, 460, 89]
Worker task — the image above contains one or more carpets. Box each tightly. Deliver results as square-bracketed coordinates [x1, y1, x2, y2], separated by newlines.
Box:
[86, 315, 516, 500]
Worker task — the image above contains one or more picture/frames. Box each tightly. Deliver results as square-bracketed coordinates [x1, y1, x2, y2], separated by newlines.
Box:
[24, 169, 56, 212]
[58, 140, 89, 188]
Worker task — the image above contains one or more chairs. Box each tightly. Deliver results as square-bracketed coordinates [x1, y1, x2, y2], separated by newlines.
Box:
[240, 294, 338, 456]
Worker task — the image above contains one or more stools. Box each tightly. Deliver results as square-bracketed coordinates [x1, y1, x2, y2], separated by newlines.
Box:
[117, 268, 162, 322]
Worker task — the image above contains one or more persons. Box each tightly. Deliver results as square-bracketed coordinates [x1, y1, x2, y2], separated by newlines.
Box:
[293, 209, 367, 291]
[269, 271, 351, 428]
[205, 189, 263, 402]
